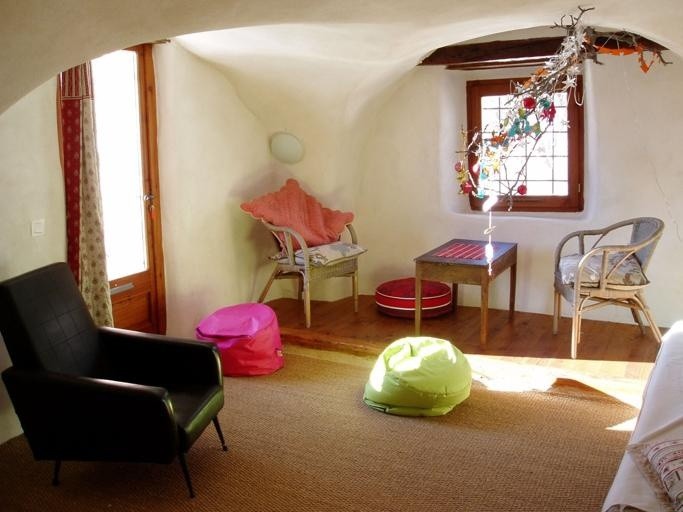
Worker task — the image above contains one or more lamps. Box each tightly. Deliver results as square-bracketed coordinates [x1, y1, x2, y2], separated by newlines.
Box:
[268, 131, 305, 165]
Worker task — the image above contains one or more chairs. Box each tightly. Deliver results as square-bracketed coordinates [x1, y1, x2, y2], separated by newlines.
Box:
[0, 263, 227, 497]
[259, 223, 359, 329]
[553, 216, 665, 360]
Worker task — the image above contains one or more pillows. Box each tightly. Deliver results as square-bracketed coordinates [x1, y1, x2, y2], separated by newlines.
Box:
[267, 241, 368, 266]
[239, 179, 354, 257]
[560, 252, 651, 290]
[629, 436, 683, 512]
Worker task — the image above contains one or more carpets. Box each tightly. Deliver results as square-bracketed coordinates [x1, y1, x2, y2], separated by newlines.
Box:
[0, 352, 639, 512]
